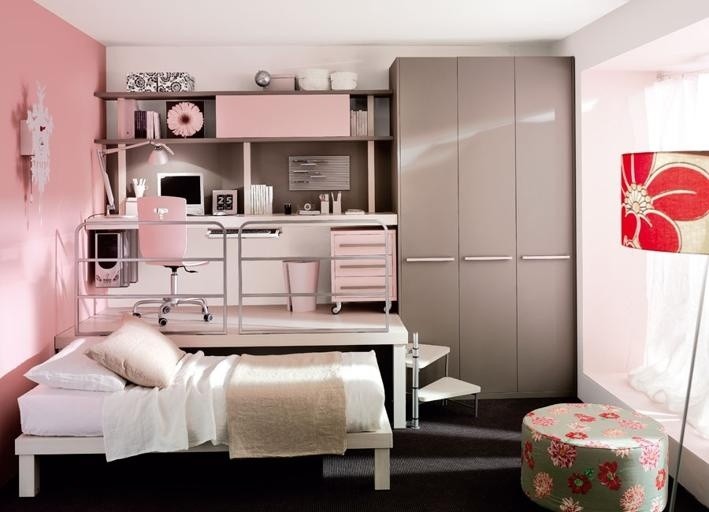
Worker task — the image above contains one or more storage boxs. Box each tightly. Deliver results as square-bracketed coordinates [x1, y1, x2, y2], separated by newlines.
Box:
[263, 72, 296, 91]
[125, 71, 195, 94]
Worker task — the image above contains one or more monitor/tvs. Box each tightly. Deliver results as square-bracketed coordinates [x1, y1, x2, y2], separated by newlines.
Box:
[157, 172, 205, 216]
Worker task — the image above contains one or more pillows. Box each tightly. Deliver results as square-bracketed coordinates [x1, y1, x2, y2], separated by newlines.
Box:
[24, 313, 185, 391]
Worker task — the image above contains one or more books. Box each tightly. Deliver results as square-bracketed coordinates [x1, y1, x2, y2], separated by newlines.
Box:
[344, 209, 366, 215]
[134, 110, 162, 140]
[299, 211, 321, 215]
[350, 109, 368, 137]
[251, 184, 273, 215]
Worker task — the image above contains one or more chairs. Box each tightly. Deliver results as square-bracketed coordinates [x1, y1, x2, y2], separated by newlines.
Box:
[127, 196, 214, 327]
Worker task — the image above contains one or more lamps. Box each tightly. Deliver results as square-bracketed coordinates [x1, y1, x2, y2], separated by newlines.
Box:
[617, 150, 708, 511]
[97, 136, 174, 210]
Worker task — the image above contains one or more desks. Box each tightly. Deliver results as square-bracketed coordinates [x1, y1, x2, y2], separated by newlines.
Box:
[86, 213, 398, 315]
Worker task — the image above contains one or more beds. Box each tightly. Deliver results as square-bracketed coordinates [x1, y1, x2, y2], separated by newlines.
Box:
[10, 353, 395, 501]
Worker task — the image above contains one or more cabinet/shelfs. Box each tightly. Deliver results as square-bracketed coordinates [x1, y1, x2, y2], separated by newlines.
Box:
[387, 52, 576, 417]
[93, 89, 394, 212]
[329, 228, 398, 313]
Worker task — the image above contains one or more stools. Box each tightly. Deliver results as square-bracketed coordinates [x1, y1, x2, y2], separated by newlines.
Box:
[519, 401, 670, 512]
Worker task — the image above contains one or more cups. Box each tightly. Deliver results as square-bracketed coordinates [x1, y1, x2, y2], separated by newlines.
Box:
[134, 185, 148, 199]
[283, 202, 292, 214]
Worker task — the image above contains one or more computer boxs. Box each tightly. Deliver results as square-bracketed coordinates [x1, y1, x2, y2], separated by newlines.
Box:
[94, 232, 122, 287]
